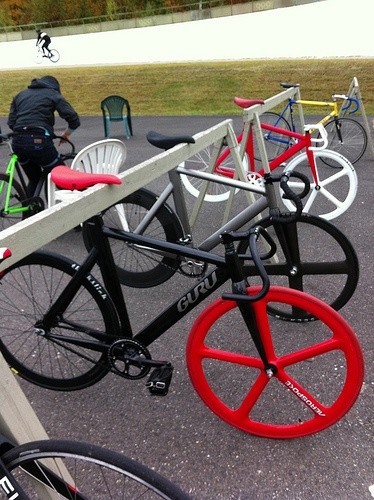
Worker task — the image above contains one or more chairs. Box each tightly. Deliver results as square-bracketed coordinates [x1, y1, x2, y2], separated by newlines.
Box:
[101, 95, 133, 140]
[47, 138, 130, 233]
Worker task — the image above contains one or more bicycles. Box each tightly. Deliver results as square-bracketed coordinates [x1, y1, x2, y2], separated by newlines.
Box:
[0, 164, 366, 441]
[249, 83, 368, 168]
[0, 441, 193, 500]
[177, 98, 359, 223]
[83, 130, 359, 324]
[1, 132, 92, 231]
[33, 43, 59, 63]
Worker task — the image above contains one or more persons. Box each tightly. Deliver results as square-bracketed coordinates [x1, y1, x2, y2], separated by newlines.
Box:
[36, 28, 54, 58]
[6, 75, 84, 232]
